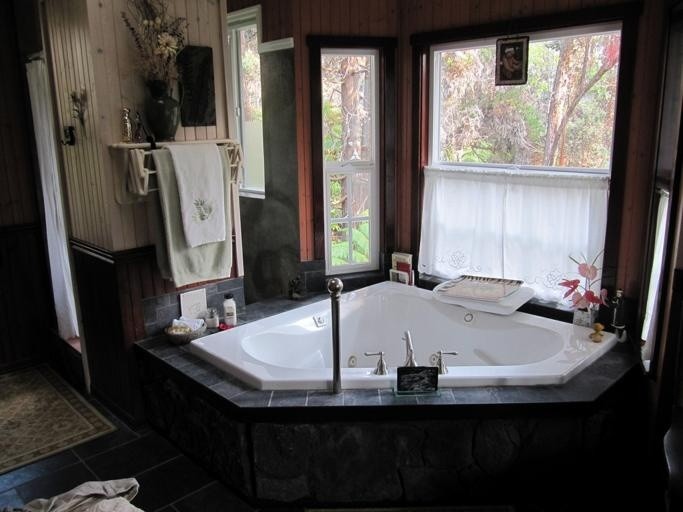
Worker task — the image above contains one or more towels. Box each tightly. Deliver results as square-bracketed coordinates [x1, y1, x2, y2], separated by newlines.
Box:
[437, 274, 524, 303]
[148, 149, 234, 289]
[162, 143, 227, 248]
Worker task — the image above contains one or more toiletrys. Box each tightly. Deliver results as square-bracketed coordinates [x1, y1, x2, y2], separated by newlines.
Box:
[132, 115, 145, 142]
[121, 107, 132, 141]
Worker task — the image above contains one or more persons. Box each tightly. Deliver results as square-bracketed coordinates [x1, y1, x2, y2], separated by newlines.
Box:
[503, 47, 521, 80]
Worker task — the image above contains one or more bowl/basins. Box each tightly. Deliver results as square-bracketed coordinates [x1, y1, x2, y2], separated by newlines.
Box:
[164, 321, 207, 345]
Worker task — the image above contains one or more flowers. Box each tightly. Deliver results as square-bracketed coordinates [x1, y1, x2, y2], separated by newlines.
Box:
[557, 249, 611, 311]
[120, 0, 188, 114]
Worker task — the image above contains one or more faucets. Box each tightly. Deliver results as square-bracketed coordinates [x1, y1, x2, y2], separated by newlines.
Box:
[402, 330, 418, 368]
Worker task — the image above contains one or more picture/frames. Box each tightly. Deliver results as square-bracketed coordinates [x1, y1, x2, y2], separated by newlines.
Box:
[495, 36, 529, 86]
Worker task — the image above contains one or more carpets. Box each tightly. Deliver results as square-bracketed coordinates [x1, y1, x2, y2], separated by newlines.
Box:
[0, 363, 119, 477]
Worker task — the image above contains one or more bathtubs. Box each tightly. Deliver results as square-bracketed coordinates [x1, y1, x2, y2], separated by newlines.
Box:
[189, 280, 617, 390]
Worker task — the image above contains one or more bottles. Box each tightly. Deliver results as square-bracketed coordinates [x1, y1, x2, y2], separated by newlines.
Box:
[611, 290, 627, 343]
[122, 107, 132, 143]
[205, 308, 219, 328]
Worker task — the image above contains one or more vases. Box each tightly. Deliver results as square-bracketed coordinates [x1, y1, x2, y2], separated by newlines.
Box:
[573, 311, 591, 351]
[146, 80, 180, 141]
